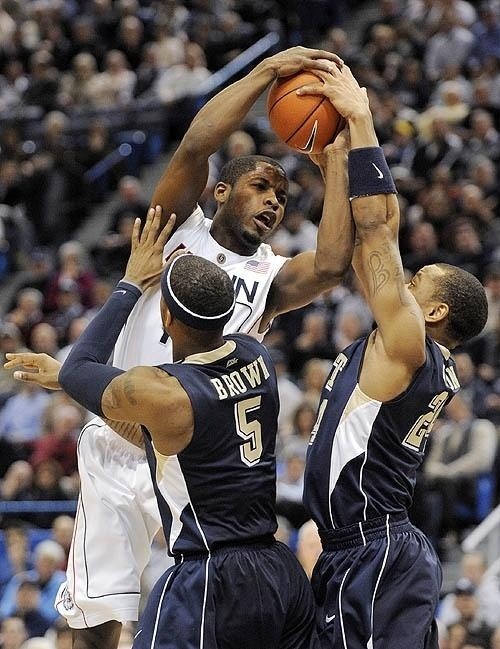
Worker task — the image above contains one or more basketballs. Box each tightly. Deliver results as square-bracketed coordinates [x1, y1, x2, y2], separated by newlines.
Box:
[267, 69, 345, 155]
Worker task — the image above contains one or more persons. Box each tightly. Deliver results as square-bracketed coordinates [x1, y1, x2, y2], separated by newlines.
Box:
[0, 1, 499, 649]
